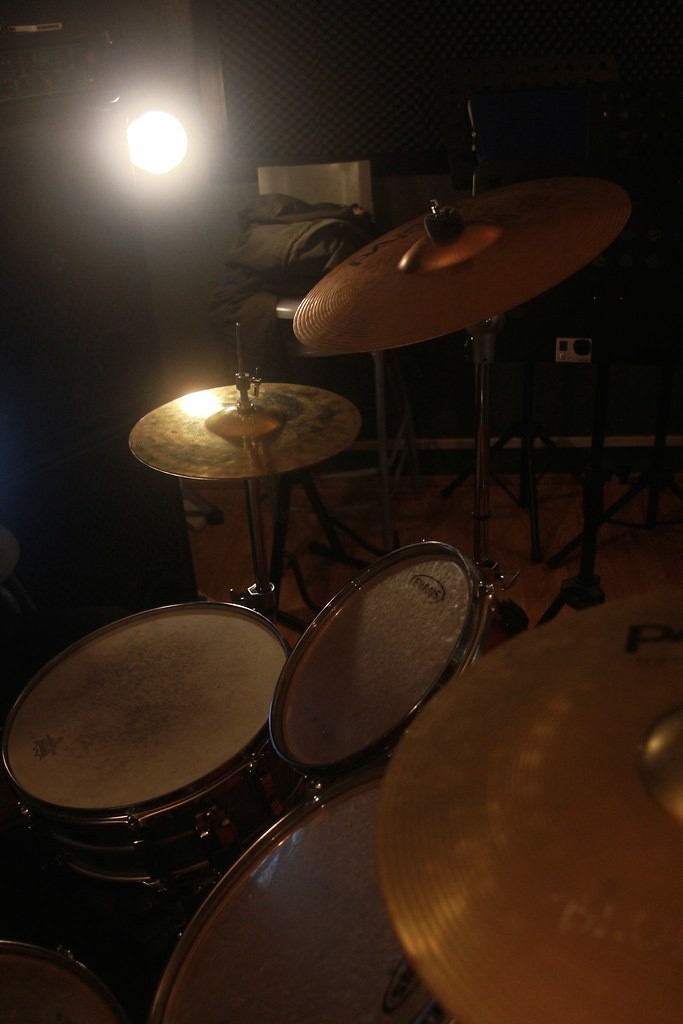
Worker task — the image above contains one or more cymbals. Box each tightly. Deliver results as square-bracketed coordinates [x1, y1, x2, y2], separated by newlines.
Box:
[128, 380, 360, 479]
[291, 177, 631, 354]
[379, 585, 683, 1024]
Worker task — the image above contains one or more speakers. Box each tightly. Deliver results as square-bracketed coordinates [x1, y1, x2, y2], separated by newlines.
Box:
[0, 394, 202, 742]
[0, 103, 161, 473]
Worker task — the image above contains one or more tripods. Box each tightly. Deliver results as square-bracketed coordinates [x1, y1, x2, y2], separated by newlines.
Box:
[440, 274, 683, 569]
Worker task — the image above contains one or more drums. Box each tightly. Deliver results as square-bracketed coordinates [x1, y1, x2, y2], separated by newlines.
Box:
[268, 541, 514, 778]
[0, 938, 131, 1024]
[1, 598, 321, 889]
[144, 755, 461, 1024]
